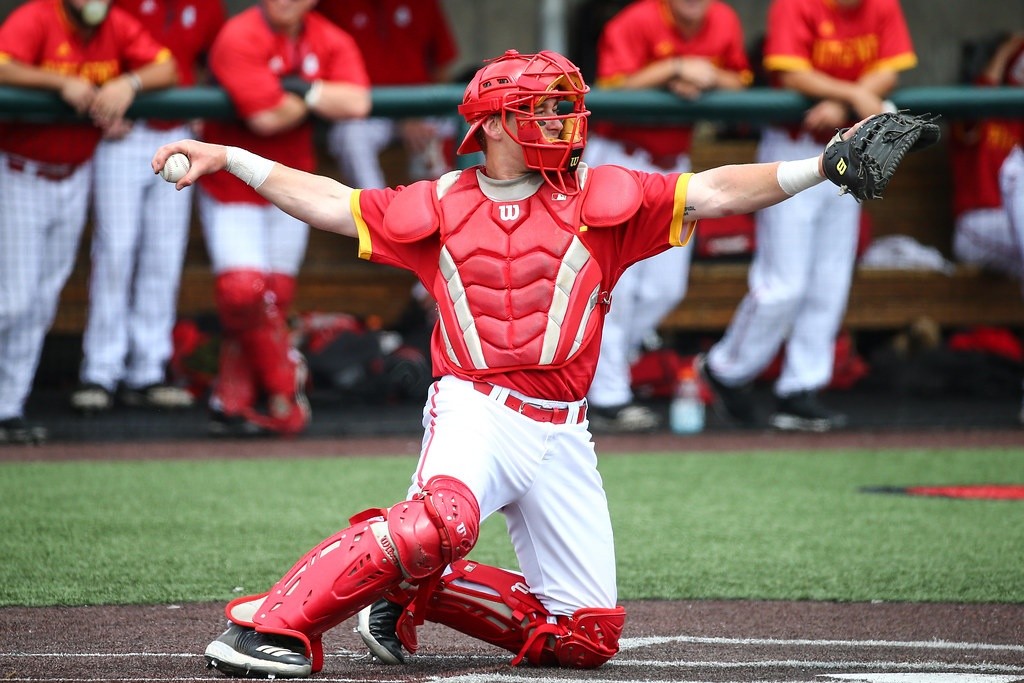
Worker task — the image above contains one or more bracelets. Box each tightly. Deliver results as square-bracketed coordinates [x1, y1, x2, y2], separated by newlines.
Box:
[224, 145, 276, 190]
[776, 157, 827, 200]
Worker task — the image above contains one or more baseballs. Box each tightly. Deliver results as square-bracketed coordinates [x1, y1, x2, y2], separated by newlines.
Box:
[158, 151, 191, 184]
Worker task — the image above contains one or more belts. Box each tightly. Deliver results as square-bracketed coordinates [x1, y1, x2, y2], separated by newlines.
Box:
[788, 128, 838, 144]
[624, 145, 679, 170]
[473, 380, 586, 424]
[7, 153, 77, 179]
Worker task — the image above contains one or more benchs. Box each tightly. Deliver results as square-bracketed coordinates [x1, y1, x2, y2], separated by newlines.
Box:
[48, 130, 1024, 330]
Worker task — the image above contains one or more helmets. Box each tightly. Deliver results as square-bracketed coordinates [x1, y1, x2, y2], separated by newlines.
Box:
[457, 48, 565, 155]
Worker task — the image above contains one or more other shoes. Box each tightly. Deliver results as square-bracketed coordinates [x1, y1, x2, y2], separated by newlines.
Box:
[72, 382, 115, 413]
[586, 407, 661, 434]
[770, 390, 849, 434]
[121, 383, 195, 407]
[691, 356, 755, 433]
[0, 417, 48, 446]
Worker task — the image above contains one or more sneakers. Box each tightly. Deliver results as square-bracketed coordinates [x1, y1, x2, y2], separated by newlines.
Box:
[204, 625, 312, 679]
[206, 348, 309, 436]
[358, 598, 405, 665]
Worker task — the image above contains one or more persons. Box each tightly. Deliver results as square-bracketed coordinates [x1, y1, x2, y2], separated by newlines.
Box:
[315, 0, 462, 190]
[68, 0, 226, 413]
[580, 0, 752, 431]
[151, 49, 940, 676]
[0, 0, 179, 443]
[693, 0, 919, 432]
[955, 33, 1024, 269]
[196, 0, 371, 430]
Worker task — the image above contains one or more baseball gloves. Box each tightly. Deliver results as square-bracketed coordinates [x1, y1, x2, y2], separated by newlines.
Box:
[821, 108, 945, 206]
